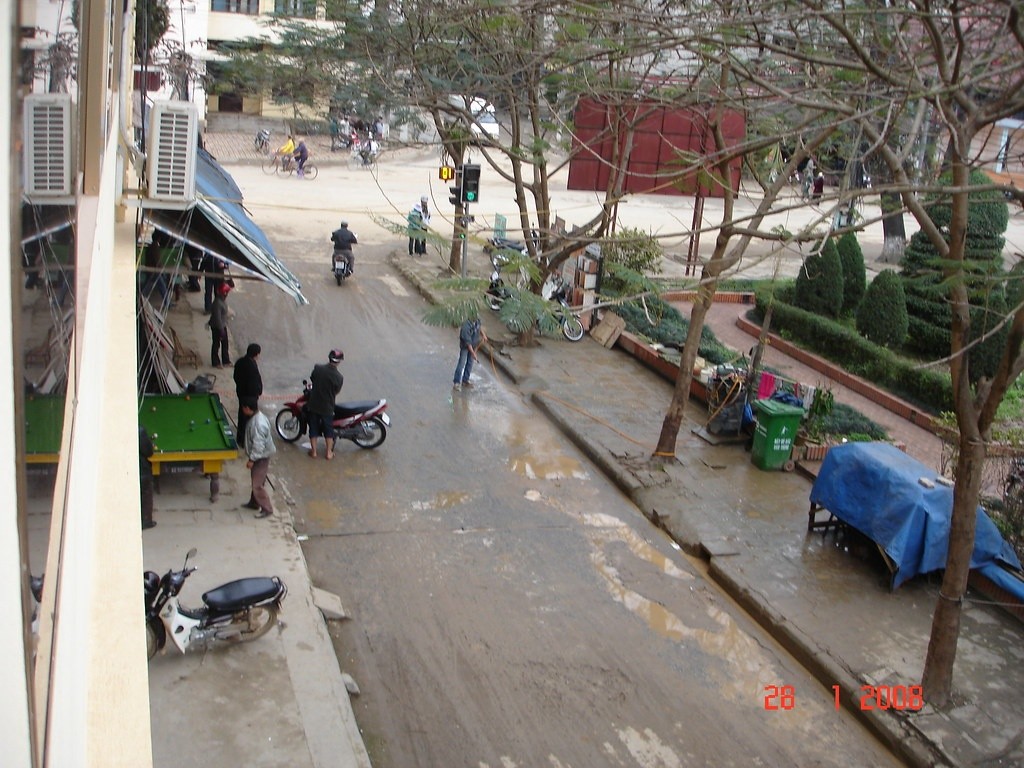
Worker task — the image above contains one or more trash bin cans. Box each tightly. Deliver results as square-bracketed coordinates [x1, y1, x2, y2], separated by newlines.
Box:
[752, 396, 808, 471]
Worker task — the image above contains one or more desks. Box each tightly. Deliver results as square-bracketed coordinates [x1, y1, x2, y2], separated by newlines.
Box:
[137, 392, 237, 502]
[20, 394, 66, 465]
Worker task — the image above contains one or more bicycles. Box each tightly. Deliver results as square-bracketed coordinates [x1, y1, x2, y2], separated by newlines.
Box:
[346, 149, 375, 172]
[254, 128, 318, 181]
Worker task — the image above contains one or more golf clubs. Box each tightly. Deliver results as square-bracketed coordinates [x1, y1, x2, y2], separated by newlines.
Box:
[206, 419, 211, 424]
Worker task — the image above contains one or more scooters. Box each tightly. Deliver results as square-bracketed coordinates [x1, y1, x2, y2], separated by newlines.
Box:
[480, 252, 585, 342]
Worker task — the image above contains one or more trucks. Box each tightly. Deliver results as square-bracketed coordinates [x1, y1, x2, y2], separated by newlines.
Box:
[443, 93, 502, 147]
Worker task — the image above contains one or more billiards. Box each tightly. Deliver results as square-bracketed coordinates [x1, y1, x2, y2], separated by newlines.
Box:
[29, 395, 34, 400]
[189, 420, 195, 432]
[154, 446, 158, 450]
[153, 433, 158, 438]
[152, 406, 156, 411]
[186, 395, 190, 400]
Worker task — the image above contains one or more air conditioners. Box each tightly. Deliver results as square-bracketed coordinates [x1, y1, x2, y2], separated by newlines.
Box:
[146, 100, 199, 202]
[21, 94, 77, 195]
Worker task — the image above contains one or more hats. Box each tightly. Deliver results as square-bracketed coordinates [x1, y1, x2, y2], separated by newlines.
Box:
[413, 204, 421, 211]
[421, 196, 428, 202]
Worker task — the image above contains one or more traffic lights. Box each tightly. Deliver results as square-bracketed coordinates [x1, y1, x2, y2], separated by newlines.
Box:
[460, 164, 482, 203]
[459, 223, 467, 238]
[448, 186, 465, 208]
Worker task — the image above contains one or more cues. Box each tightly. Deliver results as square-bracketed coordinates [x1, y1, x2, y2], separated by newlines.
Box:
[266, 475, 275, 491]
[221, 401, 238, 428]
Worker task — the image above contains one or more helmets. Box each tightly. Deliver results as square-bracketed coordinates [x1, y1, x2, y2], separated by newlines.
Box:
[329, 349, 344, 362]
[216, 283, 231, 296]
[341, 220, 348, 227]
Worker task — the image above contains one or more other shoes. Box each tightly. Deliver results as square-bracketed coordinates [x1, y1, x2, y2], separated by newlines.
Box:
[143, 521, 156, 529]
[215, 365, 223, 369]
[226, 362, 233, 367]
[256, 510, 271, 517]
[241, 503, 257, 509]
[463, 381, 473, 386]
[453, 384, 460, 391]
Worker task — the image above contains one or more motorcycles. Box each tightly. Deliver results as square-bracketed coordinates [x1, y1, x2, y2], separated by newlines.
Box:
[329, 232, 359, 286]
[143, 548, 289, 664]
[274, 378, 393, 450]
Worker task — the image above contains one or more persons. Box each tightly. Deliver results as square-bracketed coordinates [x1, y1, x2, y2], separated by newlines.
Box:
[453, 309, 488, 392]
[239, 396, 276, 519]
[184, 245, 231, 316]
[801, 169, 825, 206]
[279, 133, 309, 177]
[407, 196, 431, 256]
[138, 425, 157, 527]
[329, 114, 385, 165]
[209, 281, 235, 369]
[330, 222, 358, 273]
[306, 349, 345, 459]
[233, 344, 263, 448]
